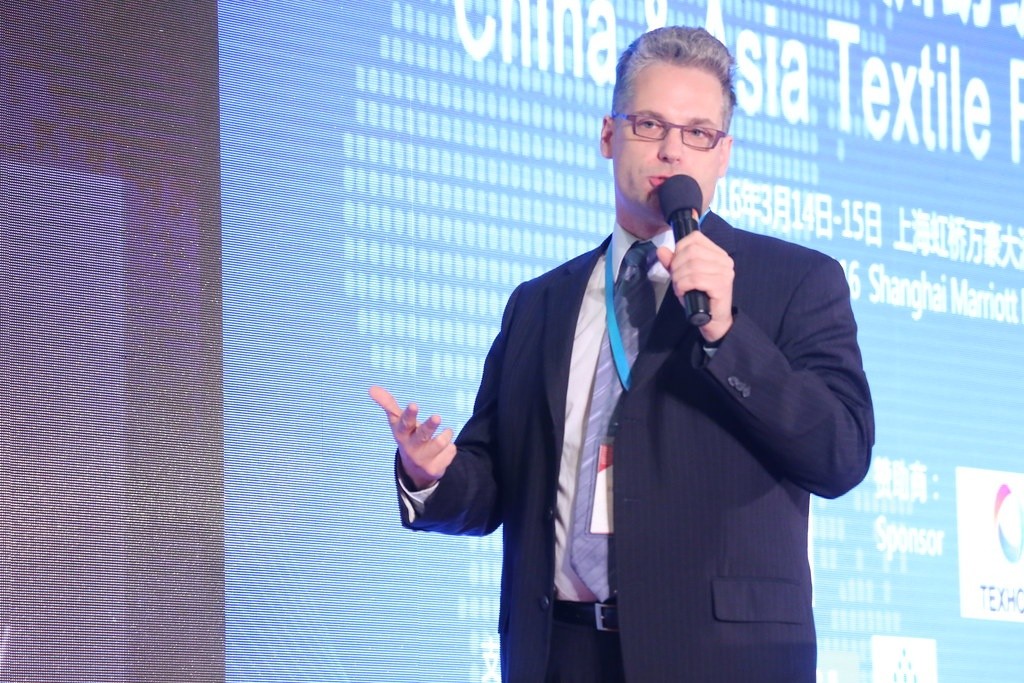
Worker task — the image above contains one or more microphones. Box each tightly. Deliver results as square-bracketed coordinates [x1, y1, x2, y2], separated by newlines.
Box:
[657, 173, 712, 326]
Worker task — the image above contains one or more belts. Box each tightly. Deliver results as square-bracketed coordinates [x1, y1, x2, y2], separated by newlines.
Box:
[552, 598, 620, 632]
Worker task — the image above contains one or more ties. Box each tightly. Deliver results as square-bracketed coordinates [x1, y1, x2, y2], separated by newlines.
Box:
[570, 241, 659, 603]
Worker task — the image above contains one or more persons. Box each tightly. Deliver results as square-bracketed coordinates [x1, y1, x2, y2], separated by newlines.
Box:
[369, 24, 874, 683]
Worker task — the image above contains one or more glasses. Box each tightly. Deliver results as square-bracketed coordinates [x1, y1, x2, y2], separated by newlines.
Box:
[611, 112, 725, 150]
